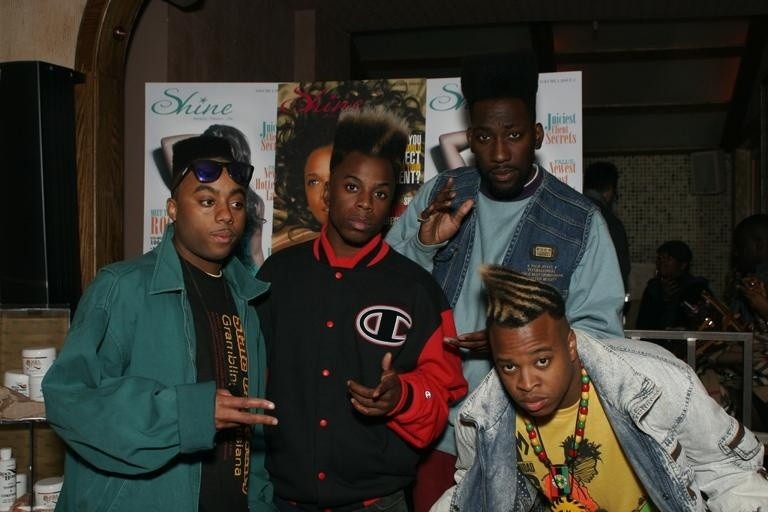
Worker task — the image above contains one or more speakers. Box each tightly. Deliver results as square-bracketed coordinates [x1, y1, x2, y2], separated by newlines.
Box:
[0, 60, 86, 309]
[690, 150, 721, 195]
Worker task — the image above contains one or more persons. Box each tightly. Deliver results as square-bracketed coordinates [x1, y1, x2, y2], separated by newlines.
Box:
[252, 106, 468, 512]
[583, 161, 631, 323]
[728, 214, 768, 332]
[273, 111, 337, 249]
[382, 59, 627, 512]
[634, 241, 710, 357]
[41, 135, 277, 512]
[439, 129, 471, 169]
[437, 264, 768, 512]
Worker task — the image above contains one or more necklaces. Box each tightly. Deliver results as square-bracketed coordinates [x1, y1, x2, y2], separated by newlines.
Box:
[519, 361, 590, 502]
[183, 257, 235, 394]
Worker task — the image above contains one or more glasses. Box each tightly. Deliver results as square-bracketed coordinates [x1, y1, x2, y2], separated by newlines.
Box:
[171, 160, 255, 188]
